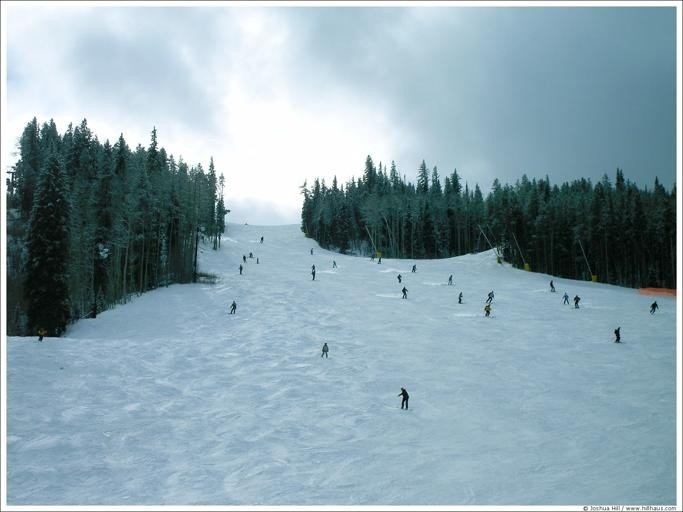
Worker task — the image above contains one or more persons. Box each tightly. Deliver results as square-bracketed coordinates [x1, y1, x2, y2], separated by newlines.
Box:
[238, 264, 242, 274]
[230, 301, 236, 314]
[484, 303, 491, 316]
[485, 290, 494, 303]
[402, 287, 408, 298]
[396, 387, 410, 410]
[457, 292, 463, 303]
[319, 343, 328, 358]
[377, 256, 381, 264]
[311, 265, 315, 269]
[614, 326, 622, 342]
[310, 248, 313, 254]
[572, 295, 581, 309]
[369, 253, 375, 262]
[332, 261, 337, 268]
[260, 236, 263, 242]
[312, 267, 315, 280]
[242, 253, 259, 265]
[411, 263, 416, 273]
[561, 292, 568, 305]
[397, 274, 402, 282]
[649, 301, 658, 315]
[549, 280, 555, 291]
[37, 327, 47, 341]
[447, 275, 452, 284]
